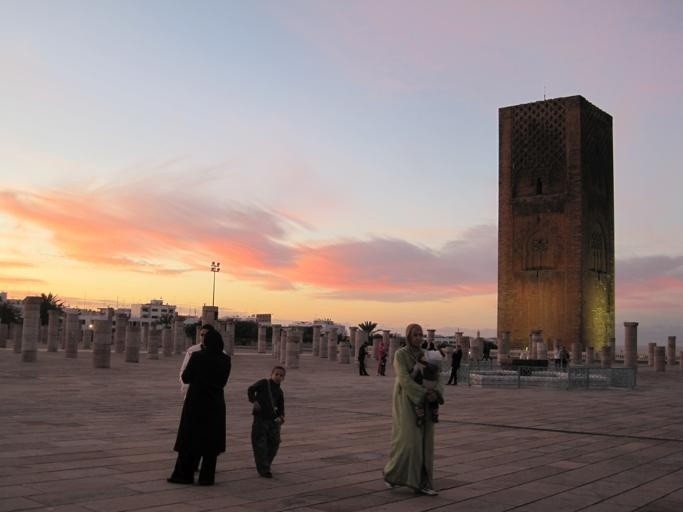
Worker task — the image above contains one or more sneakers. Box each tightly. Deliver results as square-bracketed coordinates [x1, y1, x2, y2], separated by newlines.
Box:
[166, 477, 173, 482]
[261, 473, 273, 478]
[385, 480, 394, 489]
[416, 486, 440, 496]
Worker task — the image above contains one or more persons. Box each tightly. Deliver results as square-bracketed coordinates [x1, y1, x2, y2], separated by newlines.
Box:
[376, 343, 385, 377]
[522, 344, 528, 352]
[166, 330, 230, 485]
[374, 340, 383, 372]
[357, 340, 370, 375]
[414, 343, 450, 426]
[246, 366, 285, 477]
[553, 340, 562, 367]
[178, 325, 225, 474]
[560, 346, 568, 370]
[481, 340, 491, 361]
[384, 322, 441, 495]
[444, 344, 463, 385]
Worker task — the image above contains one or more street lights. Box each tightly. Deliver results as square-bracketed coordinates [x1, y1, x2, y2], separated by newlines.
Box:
[210, 261, 220, 306]
